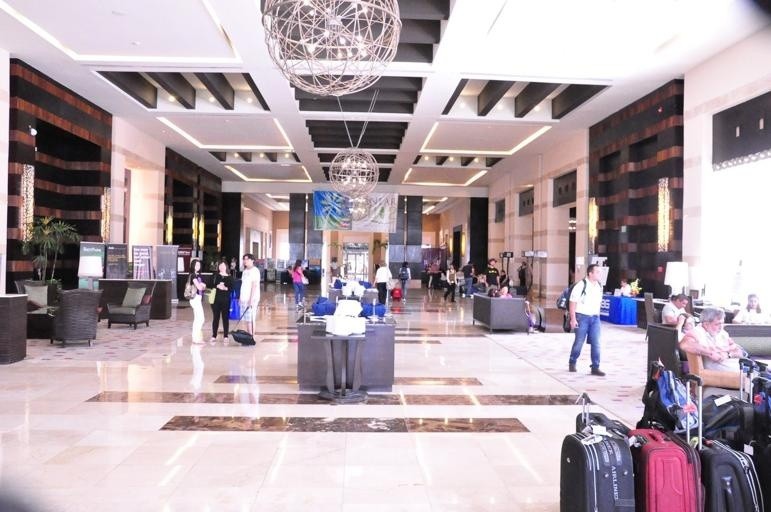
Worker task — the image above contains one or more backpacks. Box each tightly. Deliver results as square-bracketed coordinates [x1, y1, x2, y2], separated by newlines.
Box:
[556, 279, 603, 310]
[642, 364, 702, 433]
[400, 267, 408, 281]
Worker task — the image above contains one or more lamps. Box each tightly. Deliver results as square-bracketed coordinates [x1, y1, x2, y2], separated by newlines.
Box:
[262, 1, 403, 97]
[328, 89, 381, 199]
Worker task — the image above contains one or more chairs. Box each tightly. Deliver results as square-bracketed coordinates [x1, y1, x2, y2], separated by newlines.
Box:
[294, 314, 329, 400]
[471, 293, 530, 333]
[105, 281, 158, 331]
[362, 288, 379, 305]
[644, 323, 771, 384]
[14, 277, 45, 311]
[361, 320, 394, 393]
[49, 288, 102, 346]
[328, 285, 342, 301]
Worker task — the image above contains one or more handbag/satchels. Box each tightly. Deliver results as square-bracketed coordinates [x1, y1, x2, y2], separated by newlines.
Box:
[699, 394, 741, 433]
[204, 272, 217, 304]
[301, 276, 309, 285]
[231, 306, 255, 346]
[184, 274, 196, 300]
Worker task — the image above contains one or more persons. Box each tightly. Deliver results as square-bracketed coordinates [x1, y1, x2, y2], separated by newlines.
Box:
[483, 259, 500, 293]
[487, 288, 498, 297]
[619, 279, 632, 297]
[661, 294, 689, 326]
[185, 259, 205, 344]
[373, 262, 393, 306]
[518, 262, 533, 295]
[732, 294, 771, 325]
[427, 264, 436, 303]
[287, 266, 292, 274]
[499, 271, 506, 284]
[292, 260, 307, 307]
[495, 291, 536, 332]
[398, 262, 412, 301]
[240, 253, 261, 338]
[500, 285, 512, 299]
[679, 306, 754, 374]
[444, 263, 458, 302]
[567, 264, 607, 377]
[209, 260, 234, 342]
[462, 260, 474, 295]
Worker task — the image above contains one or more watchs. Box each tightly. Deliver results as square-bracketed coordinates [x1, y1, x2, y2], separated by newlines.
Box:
[726, 352, 732, 358]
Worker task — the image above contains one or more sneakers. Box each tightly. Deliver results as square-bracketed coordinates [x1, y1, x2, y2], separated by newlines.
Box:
[591, 367, 605, 377]
[568, 362, 577, 372]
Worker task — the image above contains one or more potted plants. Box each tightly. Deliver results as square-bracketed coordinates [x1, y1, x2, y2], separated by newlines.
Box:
[18, 214, 81, 298]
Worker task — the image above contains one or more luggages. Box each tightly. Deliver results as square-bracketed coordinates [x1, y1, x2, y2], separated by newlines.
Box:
[753, 360, 771, 379]
[627, 428, 706, 512]
[560, 393, 636, 511]
[736, 423, 771, 505]
[576, 412, 631, 442]
[391, 280, 403, 302]
[718, 356, 756, 440]
[752, 376, 771, 417]
[682, 374, 765, 511]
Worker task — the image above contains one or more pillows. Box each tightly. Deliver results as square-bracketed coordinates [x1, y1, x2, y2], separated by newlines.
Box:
[120, 288, 145, 307]
[24, 283, 49, 307]
